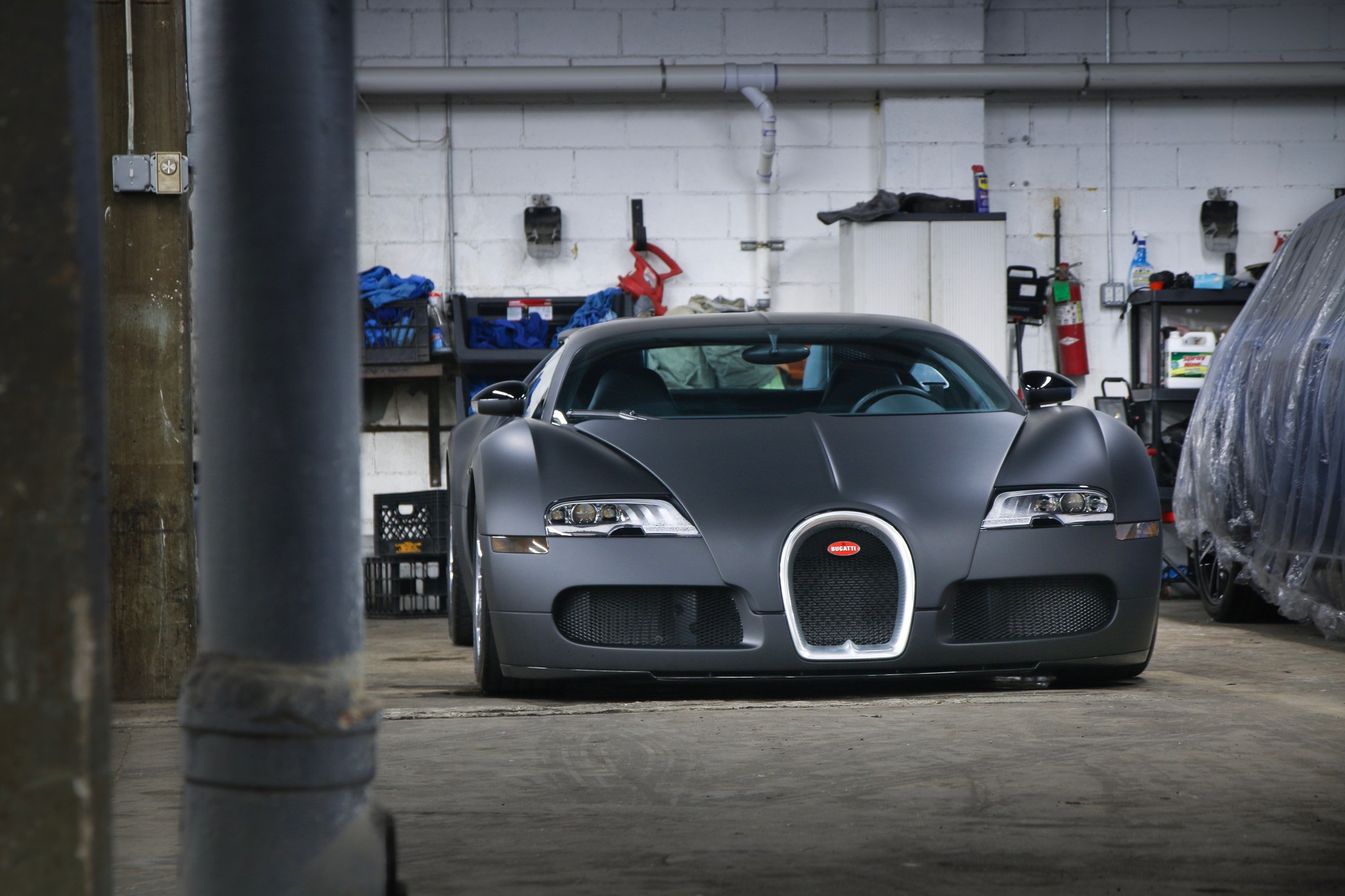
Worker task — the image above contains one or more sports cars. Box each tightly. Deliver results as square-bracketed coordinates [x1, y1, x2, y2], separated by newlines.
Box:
[448, 310, 1164, 698]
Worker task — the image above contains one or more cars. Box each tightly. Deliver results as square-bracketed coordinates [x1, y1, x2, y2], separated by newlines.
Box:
[1190, 193, 1345, 626]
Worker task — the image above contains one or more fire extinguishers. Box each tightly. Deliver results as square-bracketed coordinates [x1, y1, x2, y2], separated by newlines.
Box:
[1048, 262, 1090, 375]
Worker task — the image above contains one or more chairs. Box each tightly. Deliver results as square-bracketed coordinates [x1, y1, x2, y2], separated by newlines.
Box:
[816, 360, 902, 414]
[586, 366, 680, 417]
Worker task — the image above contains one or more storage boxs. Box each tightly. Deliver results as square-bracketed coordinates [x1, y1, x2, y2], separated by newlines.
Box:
[362, 300, 429, 366]
[362, 490, 450, 619]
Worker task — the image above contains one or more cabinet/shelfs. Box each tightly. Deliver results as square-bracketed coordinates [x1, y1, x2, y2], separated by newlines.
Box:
[1130, 288, 1254, 497]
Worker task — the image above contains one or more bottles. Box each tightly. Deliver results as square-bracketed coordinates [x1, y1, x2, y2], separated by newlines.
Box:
[633, 291, 655, 317]
[1165, 331, 1216, 389]
[972, 165, 989, 213]
[1197, 324, 1229, 344]
